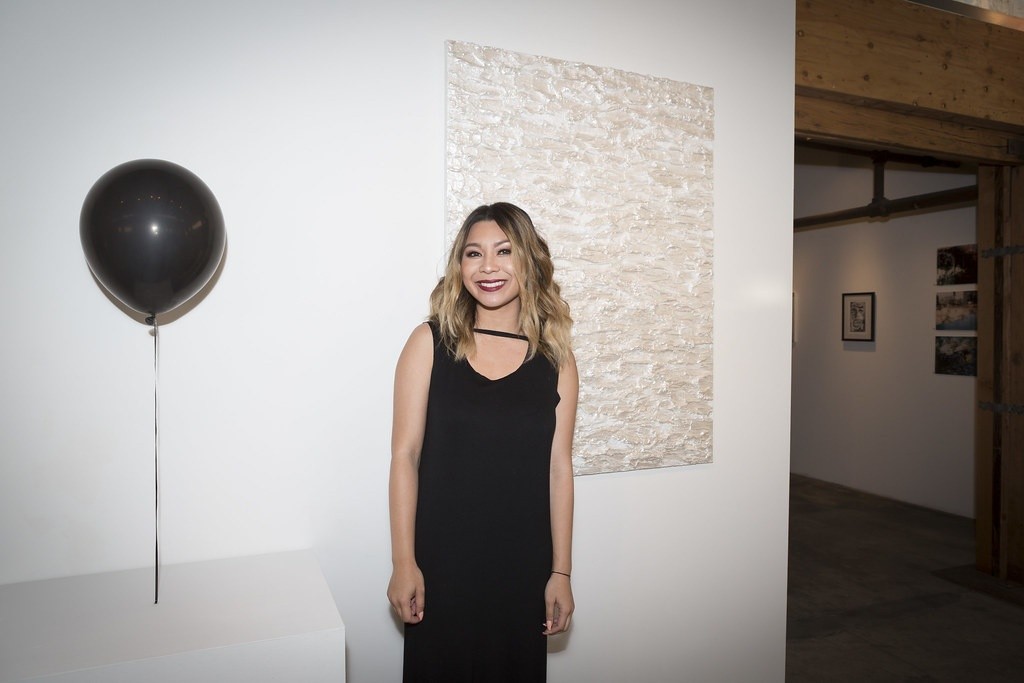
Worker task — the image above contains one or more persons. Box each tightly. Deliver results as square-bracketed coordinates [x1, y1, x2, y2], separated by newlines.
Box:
[385, 202, 578, 683]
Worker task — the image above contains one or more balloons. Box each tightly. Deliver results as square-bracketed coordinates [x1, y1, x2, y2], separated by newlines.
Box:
[80, 157, 225, 329]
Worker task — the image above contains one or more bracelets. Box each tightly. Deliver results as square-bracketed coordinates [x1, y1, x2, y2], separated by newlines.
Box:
[550, 571, 570, 577]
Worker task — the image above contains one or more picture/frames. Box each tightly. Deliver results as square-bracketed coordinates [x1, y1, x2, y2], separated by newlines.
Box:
[842, 292, 875, 342]
